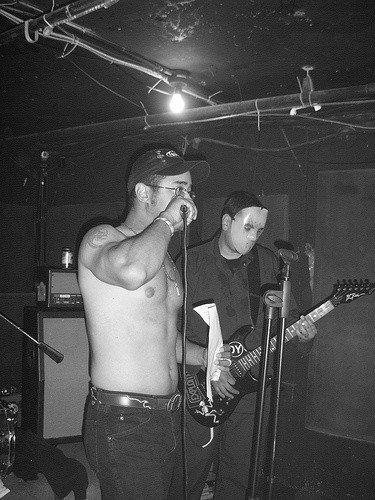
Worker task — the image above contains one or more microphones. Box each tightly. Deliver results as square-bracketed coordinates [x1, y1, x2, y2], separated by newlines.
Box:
[278, 248, 300, 261]
[180, 205, 188, 219]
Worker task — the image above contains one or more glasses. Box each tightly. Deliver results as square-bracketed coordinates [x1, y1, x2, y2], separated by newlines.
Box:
[141, 181, 198, 202]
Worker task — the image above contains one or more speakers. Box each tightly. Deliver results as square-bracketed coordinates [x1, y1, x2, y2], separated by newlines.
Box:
[24, 305, 89, 445]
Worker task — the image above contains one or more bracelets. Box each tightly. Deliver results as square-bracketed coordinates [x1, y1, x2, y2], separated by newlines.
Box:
[153, 217, 174, 237]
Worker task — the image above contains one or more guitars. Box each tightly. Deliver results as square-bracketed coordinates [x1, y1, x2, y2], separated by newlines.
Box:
[178, 279, 375, 428]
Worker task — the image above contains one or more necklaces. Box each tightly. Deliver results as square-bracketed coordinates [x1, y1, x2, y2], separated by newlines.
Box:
[120, 222, 180, 297]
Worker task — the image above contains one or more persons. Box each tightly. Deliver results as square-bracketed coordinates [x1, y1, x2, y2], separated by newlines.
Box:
[177, 192, 318, 500]
[76, 147, 232, 500]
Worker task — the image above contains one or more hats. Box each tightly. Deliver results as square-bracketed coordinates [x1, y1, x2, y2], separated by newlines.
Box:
[128, 147, 210, 193]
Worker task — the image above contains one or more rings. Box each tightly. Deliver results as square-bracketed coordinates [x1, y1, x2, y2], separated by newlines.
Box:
[226, 359, 233, 367]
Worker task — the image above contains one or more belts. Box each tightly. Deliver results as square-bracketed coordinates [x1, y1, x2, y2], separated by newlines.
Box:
[90, 386, 182, 413]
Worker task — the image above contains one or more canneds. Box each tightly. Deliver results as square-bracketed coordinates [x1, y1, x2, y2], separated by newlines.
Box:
[62, 248, 72, 269]
